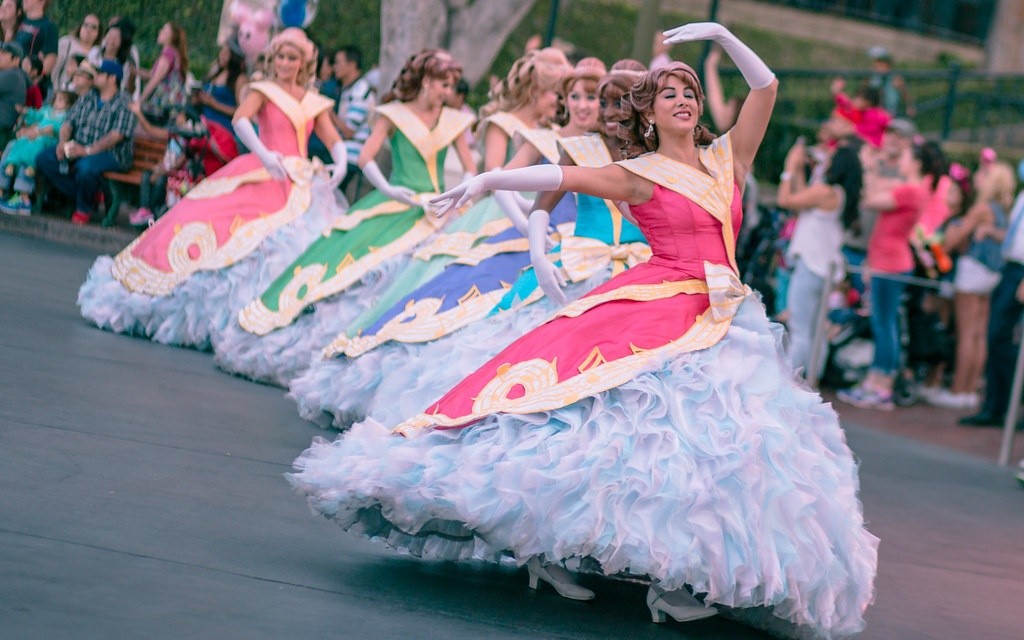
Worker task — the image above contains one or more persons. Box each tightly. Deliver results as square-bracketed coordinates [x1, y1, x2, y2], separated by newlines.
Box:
[0, 0, 250, 229]
[216, 49, 474, 389]
[705, 42, 760, 269]
[738, 48, 1024, 429]
[75, 26, 348, 352]
[290, 57, 605, 430]
[285, 21, 883, 639]
[446, 79, 478, 144]
[370, 60, 652, 423]
[310, 45, 375, 195]
[649, 28, 672, 67]
[350, 45, 572, 337]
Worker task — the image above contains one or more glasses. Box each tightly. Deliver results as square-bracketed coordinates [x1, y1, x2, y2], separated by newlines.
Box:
[82, 22, 99, 30]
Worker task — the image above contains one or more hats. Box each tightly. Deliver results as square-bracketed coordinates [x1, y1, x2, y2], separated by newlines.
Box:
[95, 61, 122, 79]
[2, 41, 25, 64]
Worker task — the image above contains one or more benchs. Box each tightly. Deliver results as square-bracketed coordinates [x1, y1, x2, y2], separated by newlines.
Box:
[99, 138, 168, 228]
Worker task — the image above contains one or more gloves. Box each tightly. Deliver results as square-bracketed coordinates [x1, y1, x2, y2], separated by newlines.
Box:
[662, 23, 775, 90]
[491, 180, 557, 252]
[429, 162, 564, 220]
[458, 171, 475, 205]
[364, 161, 422, 208]
[322, 142, 349, 190]
[529, 208, 568, 307]
[235, 116, 288, 181]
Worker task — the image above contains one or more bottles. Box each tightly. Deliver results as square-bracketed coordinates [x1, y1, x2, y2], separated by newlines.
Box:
[928, 236, 953, 273]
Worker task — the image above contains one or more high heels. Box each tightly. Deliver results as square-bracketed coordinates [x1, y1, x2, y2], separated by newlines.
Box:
[528, 555, 593, 602]
[646, 582, 718, 625]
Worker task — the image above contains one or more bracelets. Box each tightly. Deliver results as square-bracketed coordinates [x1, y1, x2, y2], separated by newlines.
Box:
[779, 172, 790, 180]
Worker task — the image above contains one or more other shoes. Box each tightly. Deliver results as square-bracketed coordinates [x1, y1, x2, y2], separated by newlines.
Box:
[129, 210, 154, 226]
[929, 388, 981, 411]
[72, 211, 89, 228]
[840, 388, 898, 413]
[0, 189, 33, 217]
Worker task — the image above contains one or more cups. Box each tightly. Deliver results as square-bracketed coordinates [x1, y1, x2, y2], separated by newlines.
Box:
[63, 140, 74, 159]
